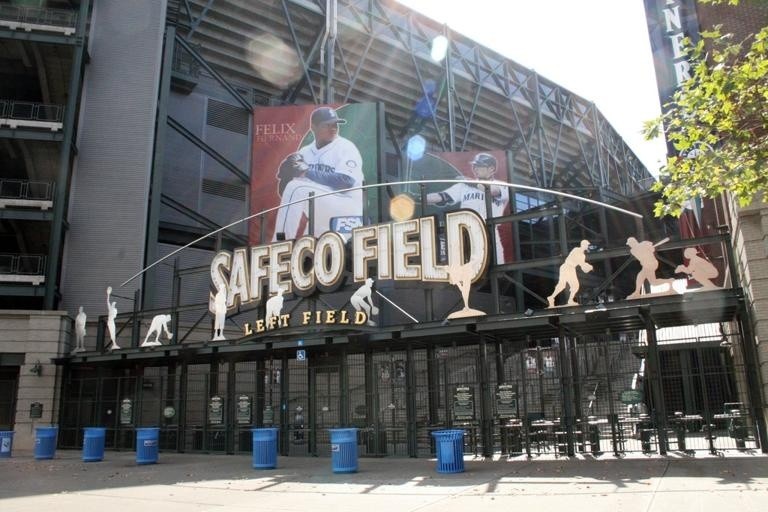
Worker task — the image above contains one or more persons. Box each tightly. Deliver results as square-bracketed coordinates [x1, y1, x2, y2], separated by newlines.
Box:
[274, 106, 365, 242]
[349, 277, 380, 327]
[141, 313, 172, 345]
[412, 151, 510, 269]
[73, 305, 86, 354]
[674, 246, 720, 292]
[265, 290, 285, 330]
[547, 238, 596, 308]
[443, 261, 475, 309]
[212, 282, 229, 341]
[625, 236, 676, 303]
[105, 292, 120, 352]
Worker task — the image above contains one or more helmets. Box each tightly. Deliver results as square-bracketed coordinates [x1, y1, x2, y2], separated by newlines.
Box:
[470, 152, 497, 170]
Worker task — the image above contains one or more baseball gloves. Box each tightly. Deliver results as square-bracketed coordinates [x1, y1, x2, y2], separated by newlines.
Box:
[280, 153, 308, 177]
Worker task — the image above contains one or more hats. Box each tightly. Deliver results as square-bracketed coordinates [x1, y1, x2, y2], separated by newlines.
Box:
[311, 108, 346, 125]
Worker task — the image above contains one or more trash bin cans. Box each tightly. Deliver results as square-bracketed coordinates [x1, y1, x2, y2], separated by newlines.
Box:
[431, 430, 466, 474]
[135, 427, 161, 465]
[327, 428, 360, 474]
[82, 427, 107, 462]
[34, 427, 59, 460]
[250, 427, 281, 470]
[0, 431, 16, 458]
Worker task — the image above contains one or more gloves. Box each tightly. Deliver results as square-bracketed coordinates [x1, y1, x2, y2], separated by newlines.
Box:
[455, 176, 480, 190]
[400, 191, 423, 203]
